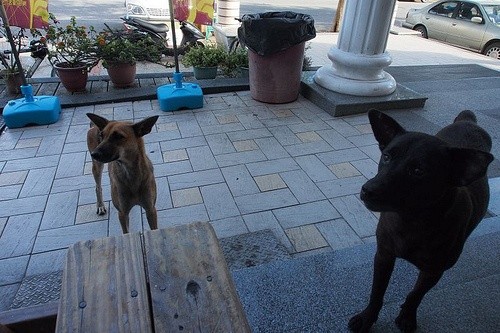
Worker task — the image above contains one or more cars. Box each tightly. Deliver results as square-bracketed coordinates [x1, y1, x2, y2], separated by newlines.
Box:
[403, 0, 500, 60]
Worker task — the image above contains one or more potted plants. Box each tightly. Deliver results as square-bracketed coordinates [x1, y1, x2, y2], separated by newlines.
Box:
[0, 18, 29, 95]
[181, 39, 250, 79]
[105, 12, 175, 86]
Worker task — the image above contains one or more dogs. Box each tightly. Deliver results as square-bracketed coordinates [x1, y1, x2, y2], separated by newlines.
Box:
[348, 109, 495, 333]
[87, 112, 160, 234]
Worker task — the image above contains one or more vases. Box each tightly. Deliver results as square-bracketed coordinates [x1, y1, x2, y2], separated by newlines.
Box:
[54, 62, 86, 93]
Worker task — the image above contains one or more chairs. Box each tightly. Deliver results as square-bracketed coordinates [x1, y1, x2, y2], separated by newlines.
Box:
[462, 5, 473, 18]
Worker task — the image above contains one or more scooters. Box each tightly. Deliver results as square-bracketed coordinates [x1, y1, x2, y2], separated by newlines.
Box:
[120, 17, 206, 61]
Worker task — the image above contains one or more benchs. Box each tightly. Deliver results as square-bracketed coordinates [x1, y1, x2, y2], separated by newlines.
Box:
[56, 221, 255, 333]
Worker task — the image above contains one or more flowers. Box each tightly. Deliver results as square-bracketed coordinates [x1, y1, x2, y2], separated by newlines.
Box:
[29, 13, 104, 66]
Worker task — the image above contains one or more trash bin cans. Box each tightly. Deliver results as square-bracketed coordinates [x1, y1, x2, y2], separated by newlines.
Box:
[238, 11, 316, 104]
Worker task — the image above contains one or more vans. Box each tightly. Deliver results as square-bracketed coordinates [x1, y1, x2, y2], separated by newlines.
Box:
[124, 0, 171, 18]
